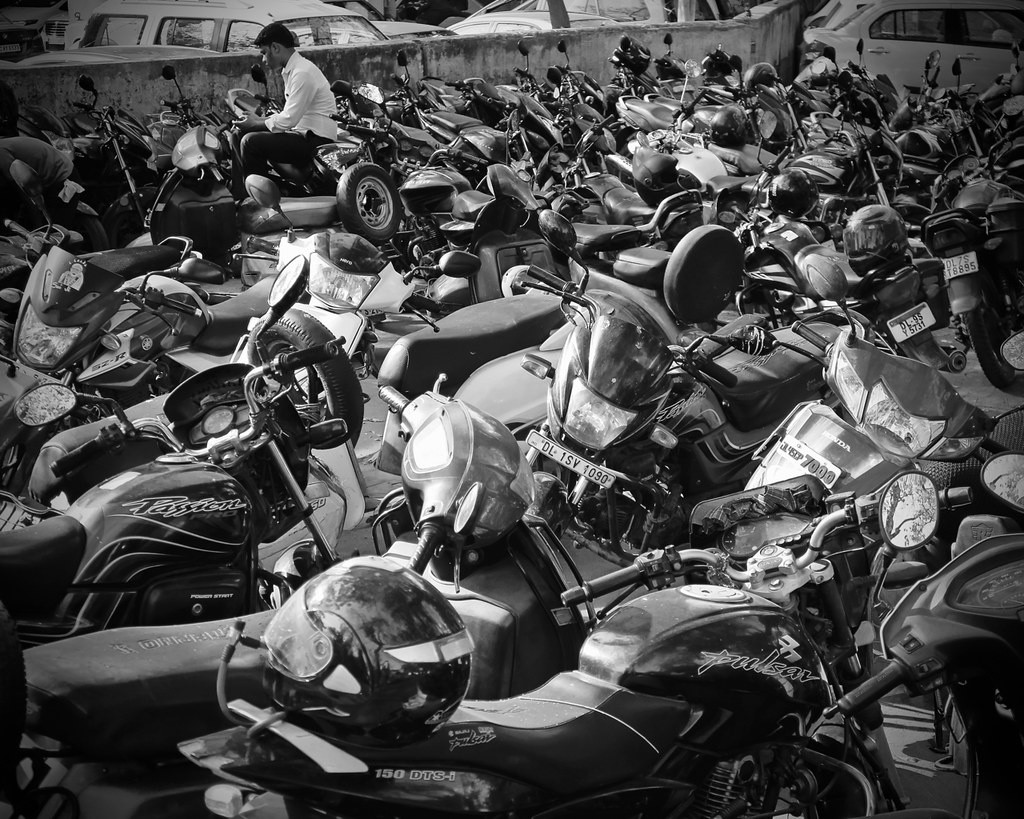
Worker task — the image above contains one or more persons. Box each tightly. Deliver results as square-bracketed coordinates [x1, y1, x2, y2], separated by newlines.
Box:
[0, 136, 77, 231]
[232, 24, 338, 197]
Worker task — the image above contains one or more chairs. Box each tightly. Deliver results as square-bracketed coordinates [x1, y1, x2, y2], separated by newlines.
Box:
[992, 30, 1014, 43]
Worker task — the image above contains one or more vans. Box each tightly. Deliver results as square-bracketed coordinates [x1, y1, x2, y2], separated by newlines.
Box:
[78, 0, 390, 54]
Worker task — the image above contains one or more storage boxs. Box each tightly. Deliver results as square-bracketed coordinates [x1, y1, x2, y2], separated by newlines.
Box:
[986, 198, 1024, 262]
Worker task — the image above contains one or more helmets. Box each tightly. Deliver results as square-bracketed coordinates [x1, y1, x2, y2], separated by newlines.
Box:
[264, 555, 471, 750]
[633, 153, 687, 208]
[746, 64, 775, 96]
[769, 167, 819, 220]
[613, 37, 651, 77]
[710, 104, 751, 147]
[843, 205, 909, 277]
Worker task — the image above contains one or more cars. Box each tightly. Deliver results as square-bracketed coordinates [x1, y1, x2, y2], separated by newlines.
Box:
[0, 0, 70, 64]
[284, 21, 459, 45]
[797, 0, 1024, 98]
[434, 10, 615, 38]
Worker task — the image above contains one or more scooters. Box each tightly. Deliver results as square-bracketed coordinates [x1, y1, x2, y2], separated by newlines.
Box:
[0, 35, 1024, 819]
